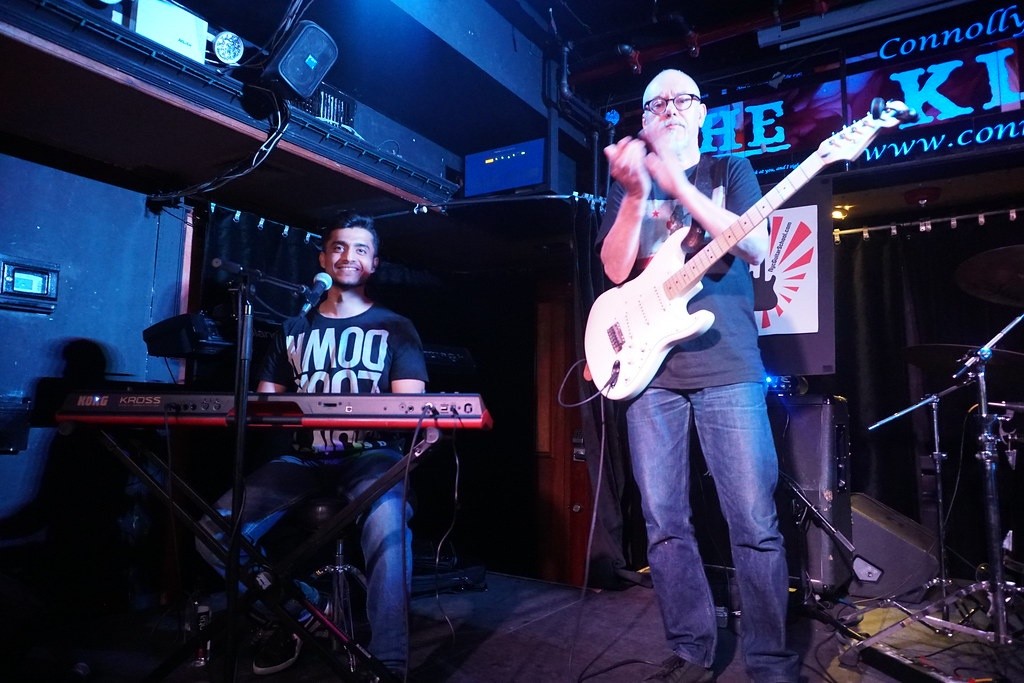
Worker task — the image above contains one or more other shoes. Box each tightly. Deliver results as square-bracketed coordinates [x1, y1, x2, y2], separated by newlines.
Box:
[252, 598, 329, 675]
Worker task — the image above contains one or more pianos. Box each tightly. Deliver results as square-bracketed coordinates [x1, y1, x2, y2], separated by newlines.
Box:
[52, 384, 496, 434]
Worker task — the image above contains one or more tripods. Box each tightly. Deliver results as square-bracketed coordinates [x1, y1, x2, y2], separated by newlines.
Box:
[773, 312, 1024, 668]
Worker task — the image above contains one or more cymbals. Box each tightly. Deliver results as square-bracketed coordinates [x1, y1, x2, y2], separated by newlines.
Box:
[952, 243, 1024, 308]
[896, 342, 1024, 372]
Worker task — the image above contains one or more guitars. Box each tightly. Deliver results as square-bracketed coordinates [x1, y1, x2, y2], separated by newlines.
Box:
[582, 99, 921, 401]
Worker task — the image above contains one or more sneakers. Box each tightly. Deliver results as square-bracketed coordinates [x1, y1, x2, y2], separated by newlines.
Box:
[641, 654, 715, 683]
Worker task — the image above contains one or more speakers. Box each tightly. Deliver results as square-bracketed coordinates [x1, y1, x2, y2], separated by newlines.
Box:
[689, 392, 855, 596]
[403, 345, 482, 572]
[849, 492, 940, 604]
[259, 19, 338, 102]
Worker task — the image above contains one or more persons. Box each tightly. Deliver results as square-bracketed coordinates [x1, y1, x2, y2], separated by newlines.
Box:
[600, 69, 800, 683]
[195, 211, 429, 683]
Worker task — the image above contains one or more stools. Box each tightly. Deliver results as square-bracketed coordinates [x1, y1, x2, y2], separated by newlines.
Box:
[290, 495, 362, 673]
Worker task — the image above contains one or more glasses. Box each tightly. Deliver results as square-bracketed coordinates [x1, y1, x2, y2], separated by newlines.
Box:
[644, 93, 701, 115]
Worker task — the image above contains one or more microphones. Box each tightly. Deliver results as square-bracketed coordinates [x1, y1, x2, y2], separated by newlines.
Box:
[299, 272, 333, 318]
